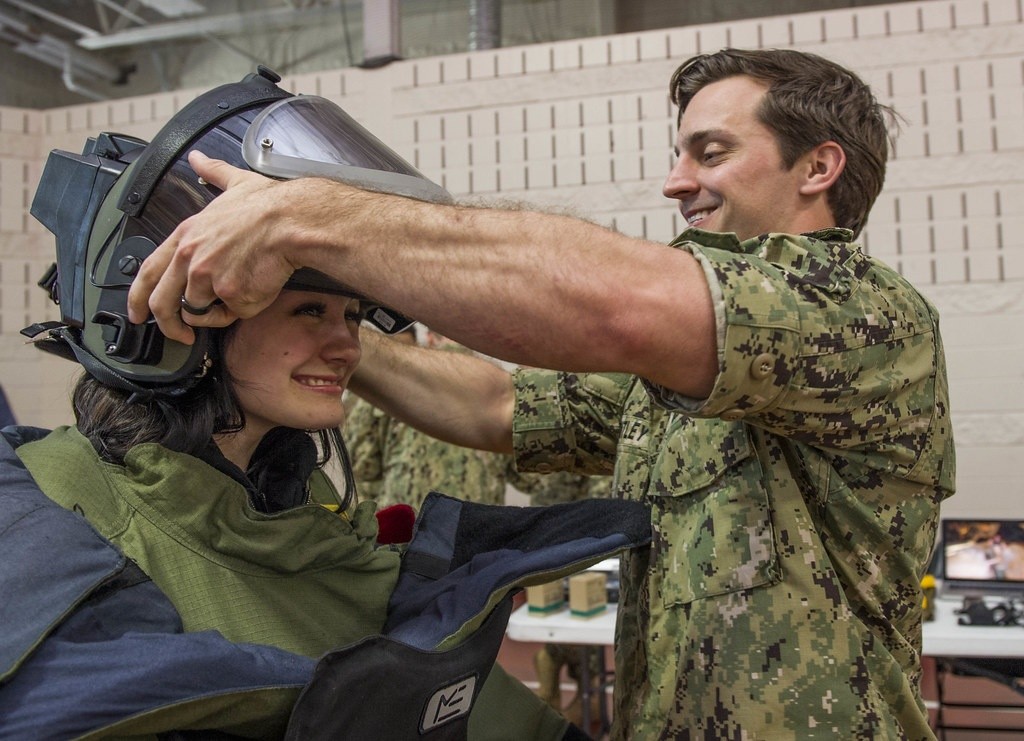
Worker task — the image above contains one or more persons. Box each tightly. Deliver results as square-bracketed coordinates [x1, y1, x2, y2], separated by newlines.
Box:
[125, 48, 958, 741]
[347, 306, 525, 552]
[337, 326, 420, 506]
[2, 72, 604, 741]
[508, 348, 617, 727]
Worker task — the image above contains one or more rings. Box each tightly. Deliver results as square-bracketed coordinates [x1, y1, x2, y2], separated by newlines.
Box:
[179, 295, 215, 316]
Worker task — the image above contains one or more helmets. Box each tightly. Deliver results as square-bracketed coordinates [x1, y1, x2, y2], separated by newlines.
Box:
[19, 66, 453, 401]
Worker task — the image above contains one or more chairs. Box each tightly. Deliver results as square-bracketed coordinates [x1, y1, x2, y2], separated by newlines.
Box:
[932, 541, 1024, 741]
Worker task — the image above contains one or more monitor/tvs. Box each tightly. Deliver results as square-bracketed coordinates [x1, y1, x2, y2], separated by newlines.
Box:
[941, 519, 1024, 591]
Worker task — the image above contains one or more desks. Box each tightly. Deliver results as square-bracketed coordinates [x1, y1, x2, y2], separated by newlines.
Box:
[507, 594, 1024, 741]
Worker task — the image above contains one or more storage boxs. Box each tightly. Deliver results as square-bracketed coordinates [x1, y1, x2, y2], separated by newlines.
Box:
[567, 571, 608, 618]
[526, 578, 567, 615]
